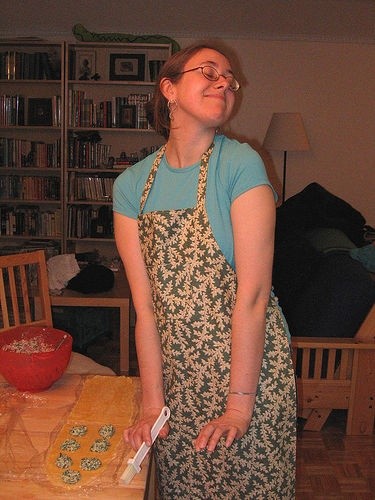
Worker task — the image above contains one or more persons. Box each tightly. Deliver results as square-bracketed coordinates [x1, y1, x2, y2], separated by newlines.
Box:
[113, 41, 298, 500]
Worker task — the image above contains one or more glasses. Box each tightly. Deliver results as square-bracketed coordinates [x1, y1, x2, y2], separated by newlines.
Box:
[173, 66, 240, 93]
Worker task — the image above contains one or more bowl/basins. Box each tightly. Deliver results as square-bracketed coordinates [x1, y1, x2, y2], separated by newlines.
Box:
[0, 326, 73, 393]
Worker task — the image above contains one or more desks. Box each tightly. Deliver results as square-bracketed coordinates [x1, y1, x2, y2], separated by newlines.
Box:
[50, 265, 131, 376]
[0, 377, 157, 500]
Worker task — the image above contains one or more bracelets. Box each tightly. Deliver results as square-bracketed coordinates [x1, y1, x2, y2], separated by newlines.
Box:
[228, 391, 257, 396]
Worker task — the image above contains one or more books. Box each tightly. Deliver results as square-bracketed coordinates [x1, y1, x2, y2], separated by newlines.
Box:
[0, 49, 59, 80]
[66, 205, 94, 239]
[0, 136, 61, 168]
[69, 172, 116, 201]
[69, 134, 138, 168]
[0, 239, 60, 287]
[0, 174, 60, 199]
[0, 91, 61, 126]
[0, 206, 61, 237]
[68, 87, 153, 130]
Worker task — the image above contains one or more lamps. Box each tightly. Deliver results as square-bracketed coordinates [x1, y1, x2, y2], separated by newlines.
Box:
[262, 111, 311, 211]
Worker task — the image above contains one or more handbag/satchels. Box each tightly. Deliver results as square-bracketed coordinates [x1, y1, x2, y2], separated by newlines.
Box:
[66, 263, 115, 294]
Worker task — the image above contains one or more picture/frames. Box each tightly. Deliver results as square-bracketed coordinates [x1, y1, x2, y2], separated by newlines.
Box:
[109, 53, 146, 82]
[76, 50, 95, 79]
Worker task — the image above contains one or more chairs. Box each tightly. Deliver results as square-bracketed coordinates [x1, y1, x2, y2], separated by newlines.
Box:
[0, 250, 115, 377]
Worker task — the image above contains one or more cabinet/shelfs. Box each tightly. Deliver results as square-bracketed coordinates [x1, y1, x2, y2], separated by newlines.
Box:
[1, 42, 63, 253]
[66, 44, 172, 253]
